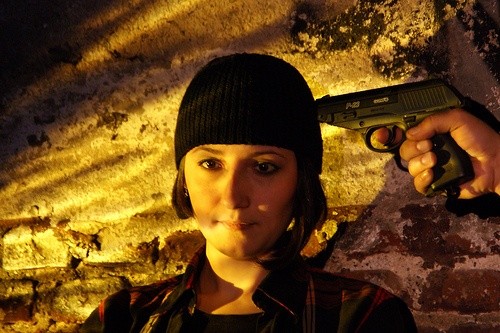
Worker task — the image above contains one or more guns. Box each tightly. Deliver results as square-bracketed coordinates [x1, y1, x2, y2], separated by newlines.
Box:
[313, 75, 474, 199]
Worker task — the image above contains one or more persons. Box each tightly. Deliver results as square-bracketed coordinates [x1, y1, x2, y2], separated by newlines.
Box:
[77, 53, 417, 333]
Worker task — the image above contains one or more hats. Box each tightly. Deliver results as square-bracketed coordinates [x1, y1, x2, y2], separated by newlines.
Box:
[175, 54, 322, 175]
